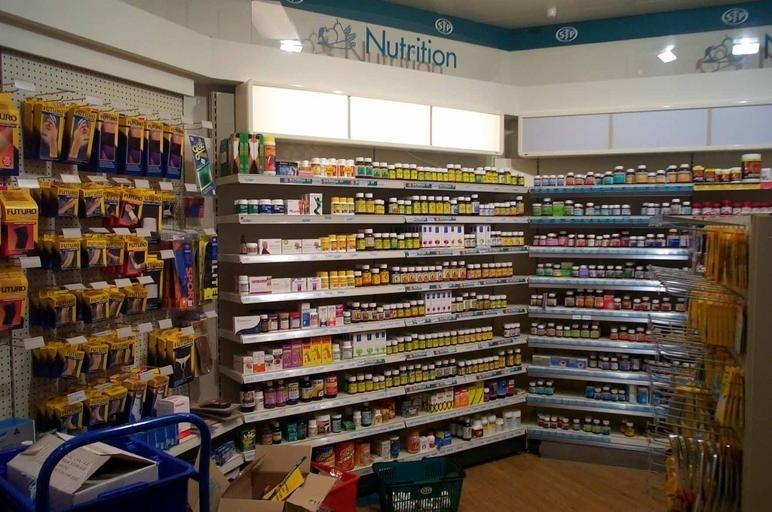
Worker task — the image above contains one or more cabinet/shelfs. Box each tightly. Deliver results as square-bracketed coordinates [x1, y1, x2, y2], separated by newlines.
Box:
[213, 173, 522, 477]
[523, 172, 772, 480]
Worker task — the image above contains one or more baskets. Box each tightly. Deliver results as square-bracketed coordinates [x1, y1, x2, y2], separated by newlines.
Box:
[372, 461, 466, 511]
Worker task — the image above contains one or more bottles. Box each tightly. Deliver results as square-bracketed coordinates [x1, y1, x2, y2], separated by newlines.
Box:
[223, 131, 522, 462]
[531, 154, 768, 439]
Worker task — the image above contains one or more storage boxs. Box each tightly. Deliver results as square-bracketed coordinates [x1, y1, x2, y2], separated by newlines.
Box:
[6, 430, 160, 506]
[188, 444, 336, 511]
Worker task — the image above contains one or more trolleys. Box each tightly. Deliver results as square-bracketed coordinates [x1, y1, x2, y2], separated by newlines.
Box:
[2, 412, 210, 511]
